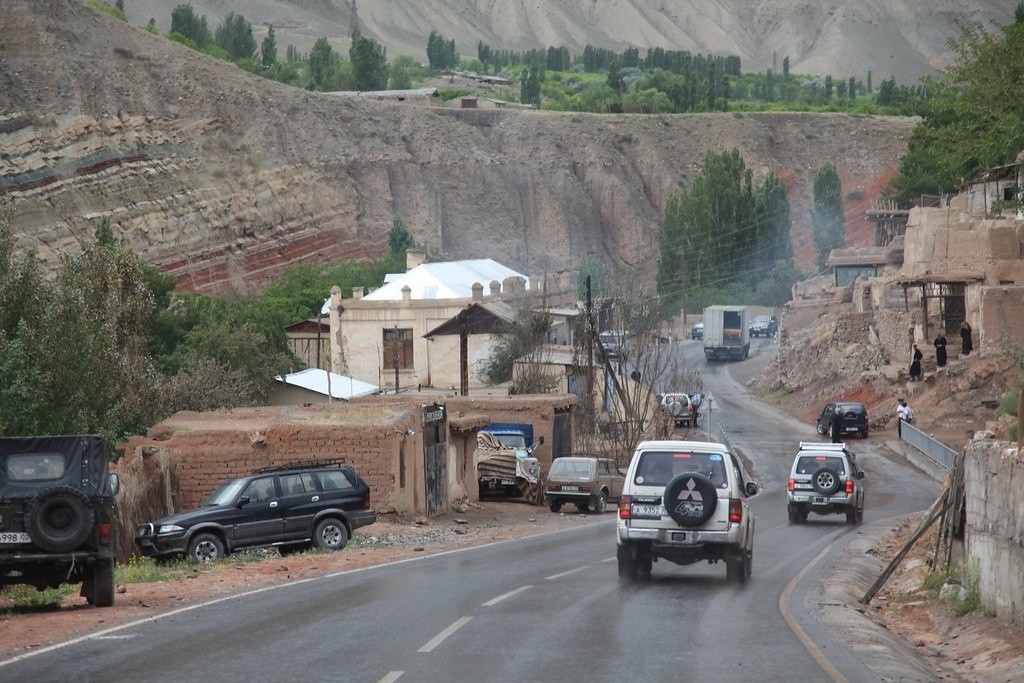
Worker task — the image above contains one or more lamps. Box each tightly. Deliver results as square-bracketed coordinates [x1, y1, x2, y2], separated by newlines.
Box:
[406, 428, 415, 437]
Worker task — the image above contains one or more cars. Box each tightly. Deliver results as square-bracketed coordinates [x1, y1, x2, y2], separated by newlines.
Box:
[691, 323, 703, 339]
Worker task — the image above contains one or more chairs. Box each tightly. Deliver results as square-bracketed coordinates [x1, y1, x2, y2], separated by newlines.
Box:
[265, 487, 275, 501]
[251, 489, 260, 503]
[308, 479, 317, 493]
[325, 479, 337, 491]
[292, 482, 303, 495]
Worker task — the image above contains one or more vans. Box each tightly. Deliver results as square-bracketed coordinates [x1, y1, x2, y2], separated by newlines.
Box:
[595, 330, 630, 364]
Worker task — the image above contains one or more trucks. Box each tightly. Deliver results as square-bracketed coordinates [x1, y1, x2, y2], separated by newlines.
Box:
[476, 422, 544, 505]
[703, 305, 752, 362]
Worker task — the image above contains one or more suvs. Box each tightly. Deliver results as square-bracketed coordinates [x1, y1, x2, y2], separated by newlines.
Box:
[134, 458, 376, 565]
[616, 440, 759, 588]
[786, 441, 864, 525]
[656, 393, 693, 429]
[749, 315, 778, 337]
[0, 435, 119, 607]
[545, 452, 626, 514]
[817, 402, 868, 439]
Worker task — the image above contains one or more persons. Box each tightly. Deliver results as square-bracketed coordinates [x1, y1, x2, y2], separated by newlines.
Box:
[961, 322, 973, 355]
[902, 402, 913, 423]
[934, 333, 947, 368]
[691, 392, 705, 427]
[897, 399, 908, 440]
[908, 344, 923, 381]
[830, 408, 843, 443]
